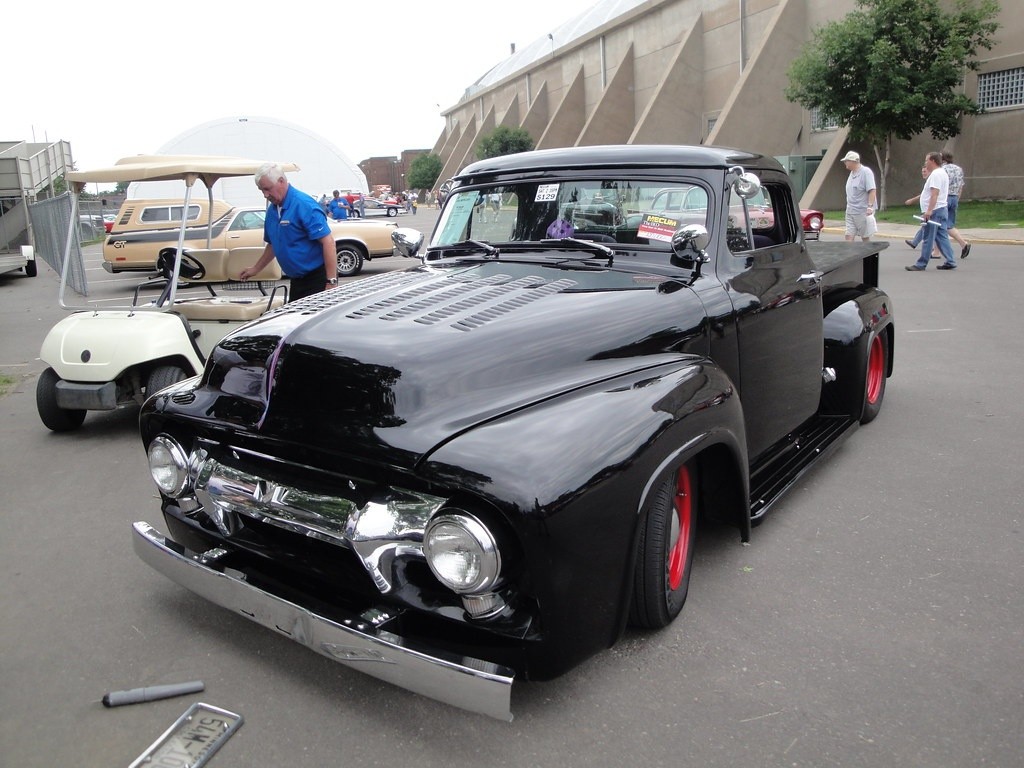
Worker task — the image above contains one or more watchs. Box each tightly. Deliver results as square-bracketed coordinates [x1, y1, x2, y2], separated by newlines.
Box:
[326, 277, 337, 284]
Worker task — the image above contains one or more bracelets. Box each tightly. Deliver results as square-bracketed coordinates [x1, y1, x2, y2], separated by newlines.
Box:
[868, 204, 873, 207]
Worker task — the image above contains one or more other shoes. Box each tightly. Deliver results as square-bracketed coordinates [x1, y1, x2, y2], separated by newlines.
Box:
[961, 242, 971, 259]
[937, 263, 956, 270]
[930, 254, 940, 259]
[905, 240, 917, 249]
[905, 265, 924, 271]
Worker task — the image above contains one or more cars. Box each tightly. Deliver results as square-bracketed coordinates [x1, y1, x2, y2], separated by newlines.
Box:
[326, 195, 408, 220]
[625, 186, 824, 251]
[131, 143, 896, 722]
[103, 214, 117, 233]
[78, 215, 107, 238]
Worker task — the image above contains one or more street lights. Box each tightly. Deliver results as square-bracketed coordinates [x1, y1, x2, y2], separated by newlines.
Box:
[401, 174, 405, 201]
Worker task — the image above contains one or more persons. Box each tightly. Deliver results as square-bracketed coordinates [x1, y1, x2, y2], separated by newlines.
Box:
[433, 189, 442, 209]
[489, 194, 502, 222]
[319, 194, 326, 210]
[346, 191, 355, 217]
[380, 190, 418, 214]
[240, 163, 337, 303]
[328, 190, 350, 219]
[904, 150, 971, 271]
[478, 195, 487, 223]
[840, 150, 878, 242]
[425, 189, 431, 209]
[359, 192, 365, 217]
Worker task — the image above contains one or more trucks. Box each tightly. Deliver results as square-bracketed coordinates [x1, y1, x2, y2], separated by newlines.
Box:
[102, 199, 400, 289]
[371, 185, 392, 198]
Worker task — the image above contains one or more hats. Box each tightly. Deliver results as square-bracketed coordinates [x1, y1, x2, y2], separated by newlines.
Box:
[840, 151, 861, 163]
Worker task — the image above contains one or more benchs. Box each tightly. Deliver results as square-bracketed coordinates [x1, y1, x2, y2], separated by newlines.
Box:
[143, 294, 290, 322]
[572, 229, 772, 252]
